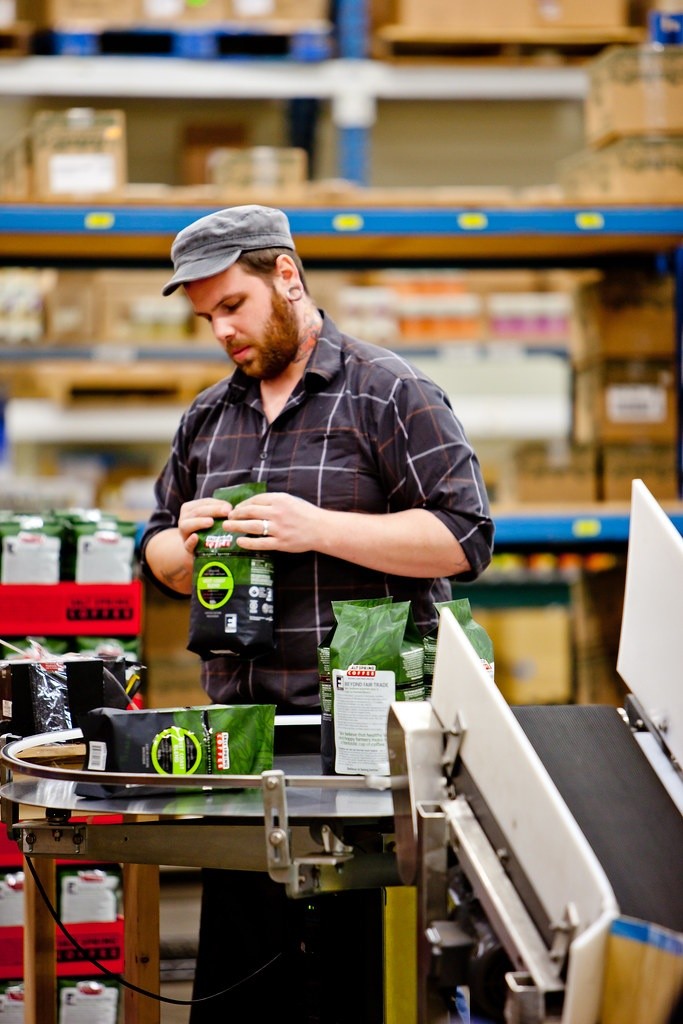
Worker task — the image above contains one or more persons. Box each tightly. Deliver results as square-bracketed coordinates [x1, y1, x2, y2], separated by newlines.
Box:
[137, 205, 496, 1024]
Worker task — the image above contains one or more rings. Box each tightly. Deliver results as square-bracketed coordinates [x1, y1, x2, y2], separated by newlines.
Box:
[262, 520, 270, 537]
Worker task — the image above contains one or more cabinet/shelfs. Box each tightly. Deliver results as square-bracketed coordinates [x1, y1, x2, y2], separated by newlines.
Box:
[0, 0, 589, 185]
[0, 203, 683, 550]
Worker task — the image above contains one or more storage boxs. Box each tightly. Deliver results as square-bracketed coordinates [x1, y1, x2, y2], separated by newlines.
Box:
[0, 0, 683, 1024]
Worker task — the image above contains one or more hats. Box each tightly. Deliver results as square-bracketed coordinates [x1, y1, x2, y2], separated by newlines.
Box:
[161, 204, 296, 297]
